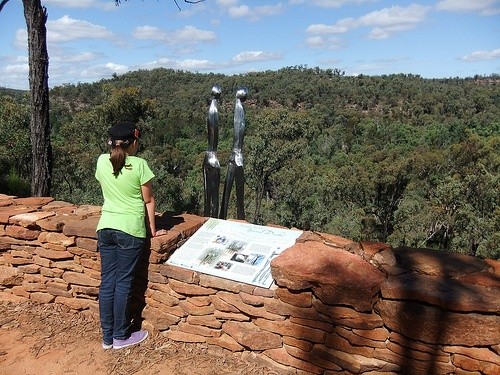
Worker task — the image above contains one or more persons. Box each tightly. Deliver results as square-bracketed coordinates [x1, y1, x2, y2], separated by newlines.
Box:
[95, 123, 167, 350]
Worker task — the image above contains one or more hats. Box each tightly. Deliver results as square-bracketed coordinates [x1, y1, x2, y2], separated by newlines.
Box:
[108, 123, 142, 148]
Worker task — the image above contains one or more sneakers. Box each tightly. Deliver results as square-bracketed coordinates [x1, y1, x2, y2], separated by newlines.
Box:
[102, 329, 149, 349]
